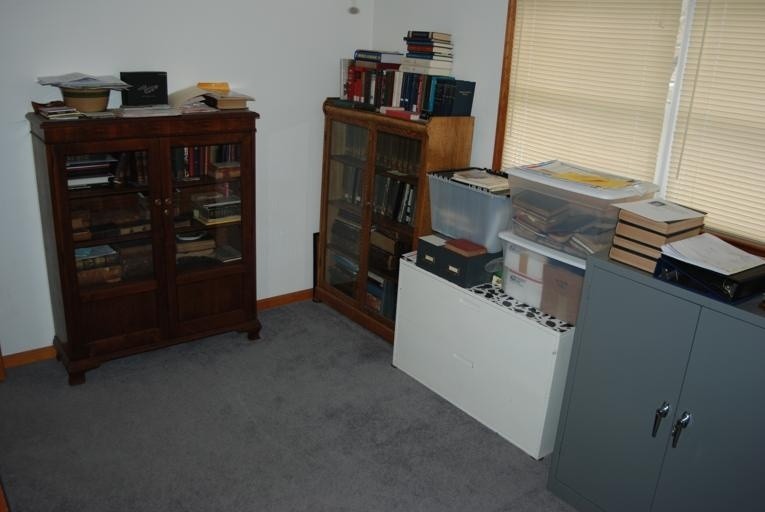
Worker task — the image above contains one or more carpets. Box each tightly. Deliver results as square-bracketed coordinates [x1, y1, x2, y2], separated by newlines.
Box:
[0, 299, 567, 512]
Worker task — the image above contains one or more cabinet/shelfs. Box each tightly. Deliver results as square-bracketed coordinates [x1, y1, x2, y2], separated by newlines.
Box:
[30, 131, 262, 387]
[545, 260, 764, 511]
[316, 102, 476, 344]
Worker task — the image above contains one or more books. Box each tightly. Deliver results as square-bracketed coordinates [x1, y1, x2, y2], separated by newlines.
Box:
[326, 31, 478, 124]
[338, 126, 421, 225]
[168, 143, 243, 264]
[66, 149, 156, 283]
[325, 217, 393, 316]
[442, 163, 764, 323]
[31, 70, 256, 120]
